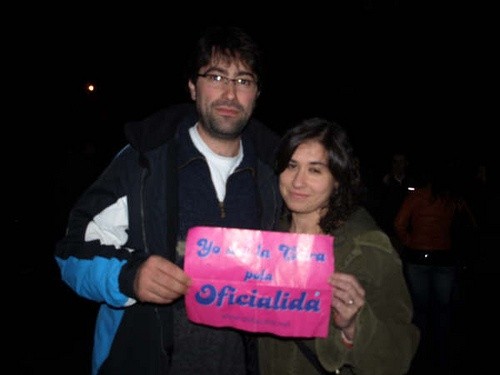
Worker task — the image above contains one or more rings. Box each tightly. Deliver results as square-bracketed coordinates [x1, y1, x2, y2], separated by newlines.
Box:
[345, 298, 356, 307]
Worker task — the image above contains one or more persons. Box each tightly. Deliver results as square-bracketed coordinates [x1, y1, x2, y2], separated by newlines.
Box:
[257, 116, 422, 375]
[54, 25, 293, 375]
[373, 150, 463, 272]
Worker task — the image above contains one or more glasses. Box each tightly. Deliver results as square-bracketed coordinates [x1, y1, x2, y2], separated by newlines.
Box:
[197, 72, 257, 91]
